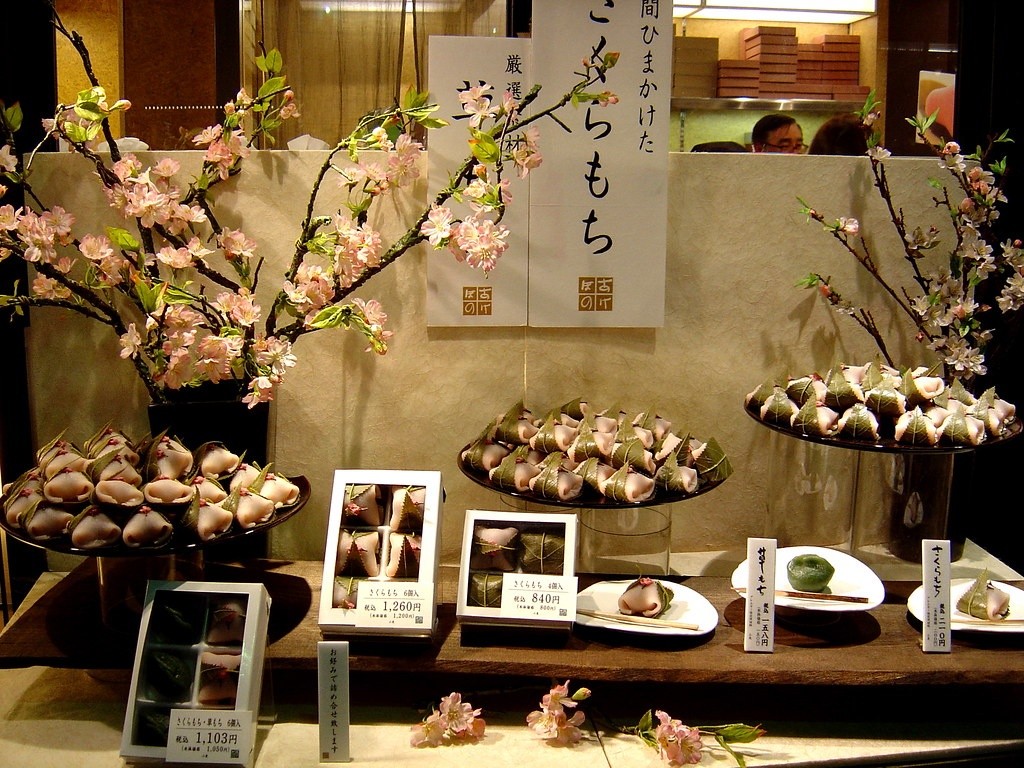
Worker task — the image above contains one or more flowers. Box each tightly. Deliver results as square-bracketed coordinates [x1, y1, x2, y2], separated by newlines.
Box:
[526, 677, 609, 745]
[0, 0, 627, 411]
[410, 691, 488, 747]
[592, 709, 766, 768]
[794, 87, 1023, 391]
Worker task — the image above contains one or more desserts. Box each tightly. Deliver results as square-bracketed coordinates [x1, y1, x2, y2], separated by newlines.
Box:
[787, 554, 835, 592]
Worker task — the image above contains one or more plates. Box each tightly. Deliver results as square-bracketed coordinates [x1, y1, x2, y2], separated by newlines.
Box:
[456, 438, 727, 510]
[0, 473, 313, 558]
[575, 578, 719, 635]
[730, 545, 886, 613]
[906, 577, 1024, 633]
[743, 394, 1024, 457]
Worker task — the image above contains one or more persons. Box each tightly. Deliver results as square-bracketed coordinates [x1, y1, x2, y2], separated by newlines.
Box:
[751, 113, 809, 155]
[808, 112, 880, 156]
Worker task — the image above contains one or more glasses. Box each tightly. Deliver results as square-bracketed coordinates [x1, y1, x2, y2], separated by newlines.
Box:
[762, 143, 809, 155]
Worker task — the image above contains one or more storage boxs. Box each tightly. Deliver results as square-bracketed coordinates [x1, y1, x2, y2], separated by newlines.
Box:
[119, 579, 272, 768]
[317, 468, 444, 637]
[671, 26, 871, 101]
[455, 510, 580, 627]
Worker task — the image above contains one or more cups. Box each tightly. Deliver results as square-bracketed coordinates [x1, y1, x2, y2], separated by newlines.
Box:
[579, 503, 673, 576]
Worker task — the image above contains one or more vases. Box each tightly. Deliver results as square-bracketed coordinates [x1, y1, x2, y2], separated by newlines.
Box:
[147, 377, 281, 560]
[888, 452, 992, 562]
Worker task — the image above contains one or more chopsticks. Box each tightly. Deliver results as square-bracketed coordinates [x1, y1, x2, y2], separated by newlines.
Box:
[576, 608, 699, 631]
